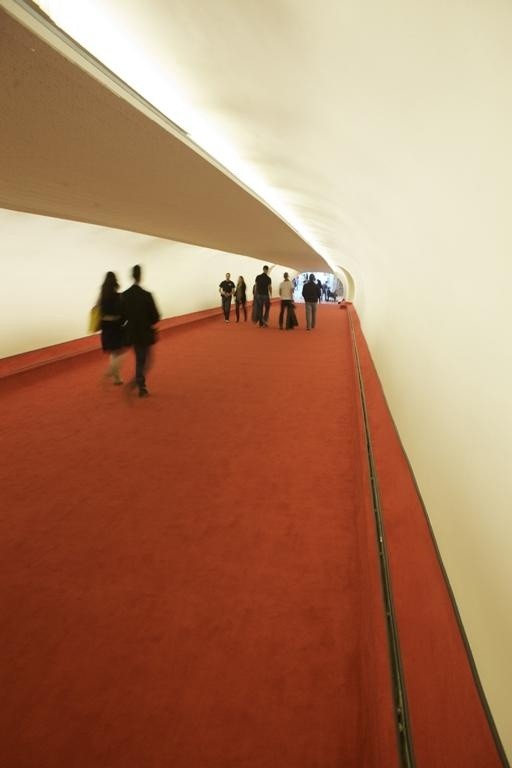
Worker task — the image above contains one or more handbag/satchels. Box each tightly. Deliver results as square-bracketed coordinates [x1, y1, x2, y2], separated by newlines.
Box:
[89, 304, 103, 330]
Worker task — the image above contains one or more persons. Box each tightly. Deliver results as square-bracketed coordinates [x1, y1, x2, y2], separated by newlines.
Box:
[219, 272, 235, 322]
[252, 282, 259, 325]
[233, 274, 248, 322]
[119, 264, 160, 398]
[96, 271, 133, 386]
[255, 265, 274, 326]
[292, 273, 339, 303]
[278, 273, 294, 330]
[303, 273, 320, 330]
